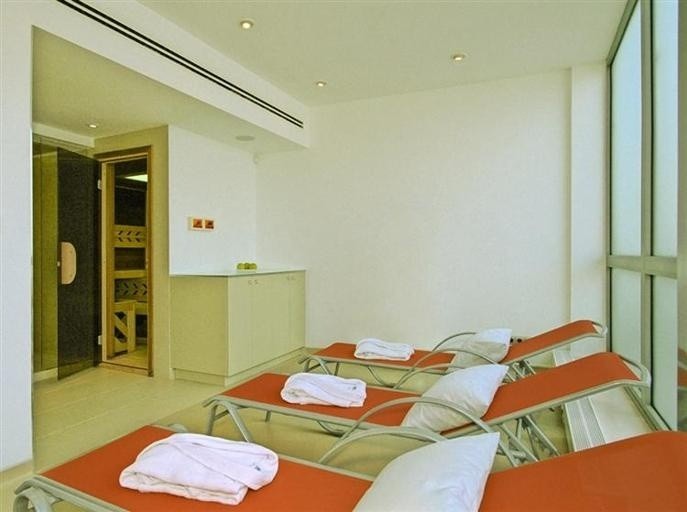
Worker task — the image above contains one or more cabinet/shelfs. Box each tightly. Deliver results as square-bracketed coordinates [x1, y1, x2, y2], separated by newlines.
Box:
[264, 270, 308, 373]
[171, 274, 264, 387]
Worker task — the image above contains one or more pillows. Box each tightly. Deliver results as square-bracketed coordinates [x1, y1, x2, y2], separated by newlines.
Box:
[352, 431, 501, 511]
[448, 328, 513, 368]
[399, 364, 510, 432]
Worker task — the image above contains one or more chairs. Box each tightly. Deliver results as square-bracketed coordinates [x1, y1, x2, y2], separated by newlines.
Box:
[14, 418, 687, 511]
[297, 319, 609, 389]
[200, 349, 653, 472]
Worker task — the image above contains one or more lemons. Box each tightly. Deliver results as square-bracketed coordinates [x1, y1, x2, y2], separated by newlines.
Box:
[249, 263, 257, 269]
[244, 262, 250, 269]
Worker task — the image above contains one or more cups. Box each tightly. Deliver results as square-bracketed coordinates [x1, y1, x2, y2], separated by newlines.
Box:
[243, 263, 249, 269]
[248, 263, 258, 270]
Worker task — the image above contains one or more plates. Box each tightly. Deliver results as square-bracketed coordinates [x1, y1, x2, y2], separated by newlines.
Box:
[231, 269, 261, 273]
